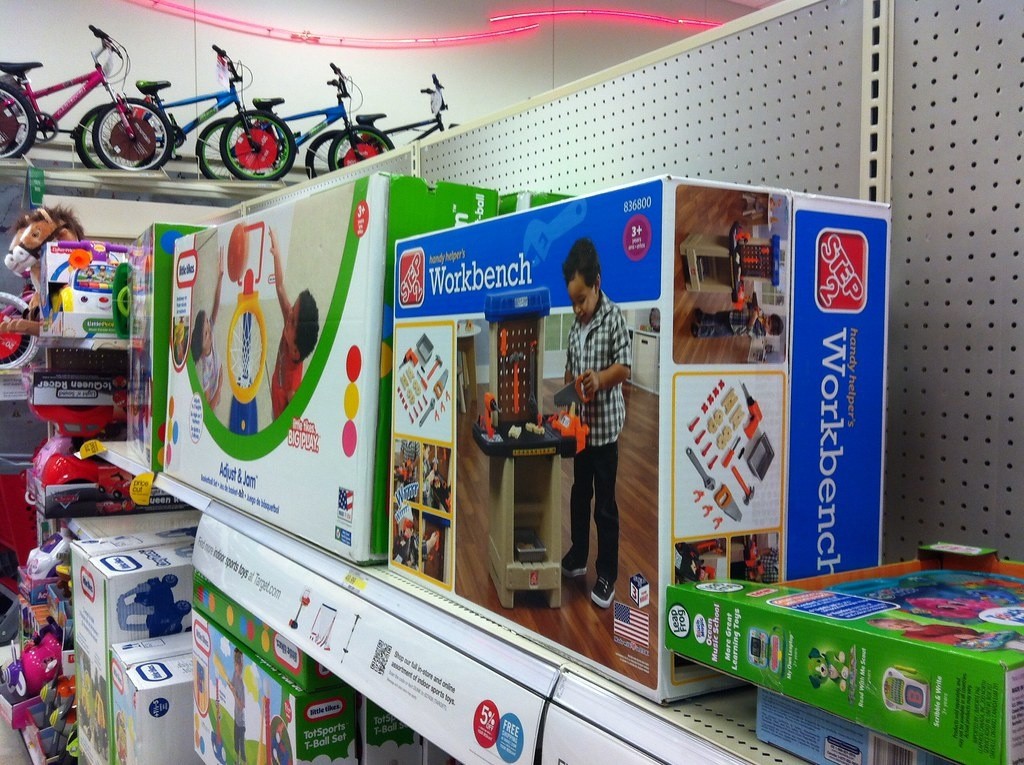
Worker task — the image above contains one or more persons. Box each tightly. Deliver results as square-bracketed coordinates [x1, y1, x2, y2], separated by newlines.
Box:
[226, 648, 247, 765]
[190, 246, 224, 412]
[867, 618, 1024, 652]
[269, 225, 320, 420]
[561, 237, 633, 608]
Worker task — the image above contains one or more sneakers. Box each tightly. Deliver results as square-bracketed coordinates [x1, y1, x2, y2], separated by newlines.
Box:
[691, 323, 699, 336]
[561, 545, 587, 578]
[590, 576, 616, 609]
[694, 306, 703, 323]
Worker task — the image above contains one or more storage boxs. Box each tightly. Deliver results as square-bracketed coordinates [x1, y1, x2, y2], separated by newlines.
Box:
[0, 167, 1024, 765]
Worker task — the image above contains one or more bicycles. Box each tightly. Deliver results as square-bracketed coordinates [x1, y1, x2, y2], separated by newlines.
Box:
[195, 62, 395, 173]
[0, 24, 175, 173]
[305, 71, 460, 180]
[0, 225, 39, 369]
[75, 43, 297, 181]
